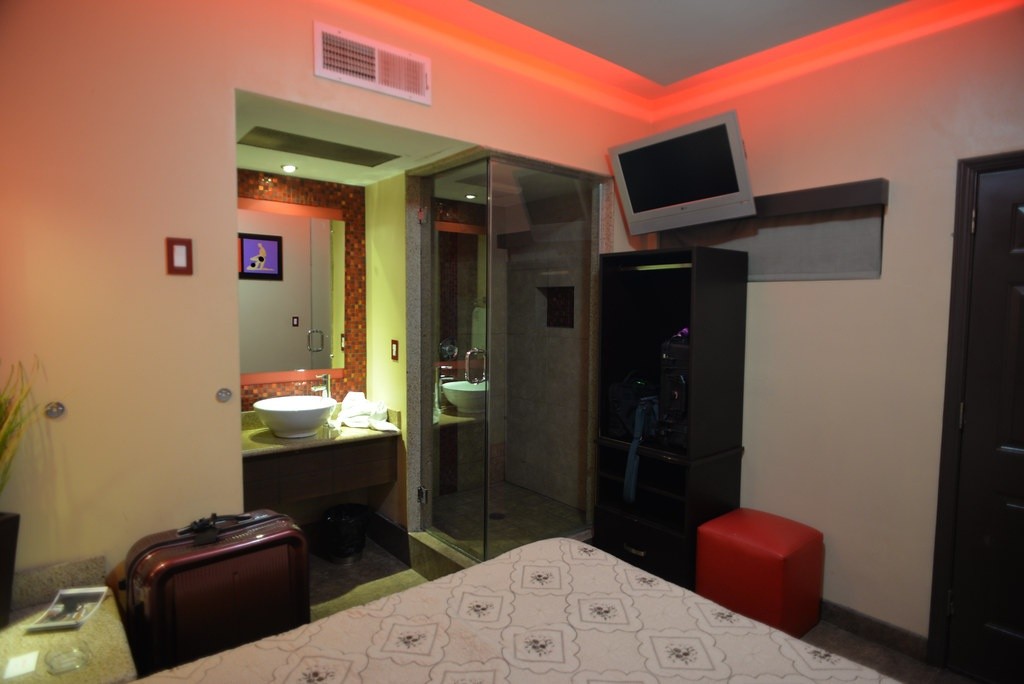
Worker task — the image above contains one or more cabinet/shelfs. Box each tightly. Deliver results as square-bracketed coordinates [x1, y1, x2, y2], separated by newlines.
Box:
[591, 242, 750, 587]
[242, 436, 398, 509]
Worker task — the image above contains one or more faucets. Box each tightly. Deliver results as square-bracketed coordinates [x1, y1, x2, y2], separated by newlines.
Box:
[310, 374, 331, 398]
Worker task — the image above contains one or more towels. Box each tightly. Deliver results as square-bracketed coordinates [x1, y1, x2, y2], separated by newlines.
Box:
[326, 390, 399, 433]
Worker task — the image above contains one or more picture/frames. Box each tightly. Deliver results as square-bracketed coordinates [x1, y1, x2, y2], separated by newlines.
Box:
[237, 231, 283, 282]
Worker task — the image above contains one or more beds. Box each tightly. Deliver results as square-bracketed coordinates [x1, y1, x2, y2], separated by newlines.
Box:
[128, 536, 901, 683]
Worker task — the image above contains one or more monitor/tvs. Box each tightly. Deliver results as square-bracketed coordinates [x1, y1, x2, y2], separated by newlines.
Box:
[605, 107, 757, 239]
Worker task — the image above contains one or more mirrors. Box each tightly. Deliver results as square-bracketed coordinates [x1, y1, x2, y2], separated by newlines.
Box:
[237, 198, 346, 377]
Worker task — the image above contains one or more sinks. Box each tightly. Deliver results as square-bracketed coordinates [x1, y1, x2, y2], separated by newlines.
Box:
[442, 380, 488, 414]
[252, 394, 338, 439]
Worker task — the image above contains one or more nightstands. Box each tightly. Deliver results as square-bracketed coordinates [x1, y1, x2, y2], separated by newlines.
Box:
[0, 582, 137, 684]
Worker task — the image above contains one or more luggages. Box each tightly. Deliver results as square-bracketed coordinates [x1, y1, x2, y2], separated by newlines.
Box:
[125, 507, 307, 668]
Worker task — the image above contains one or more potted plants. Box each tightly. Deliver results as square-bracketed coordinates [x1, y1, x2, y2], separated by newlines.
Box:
[0, 358, 53, 632]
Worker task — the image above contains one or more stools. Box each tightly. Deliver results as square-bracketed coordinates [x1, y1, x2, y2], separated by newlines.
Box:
[695, 510, 824, 640]
